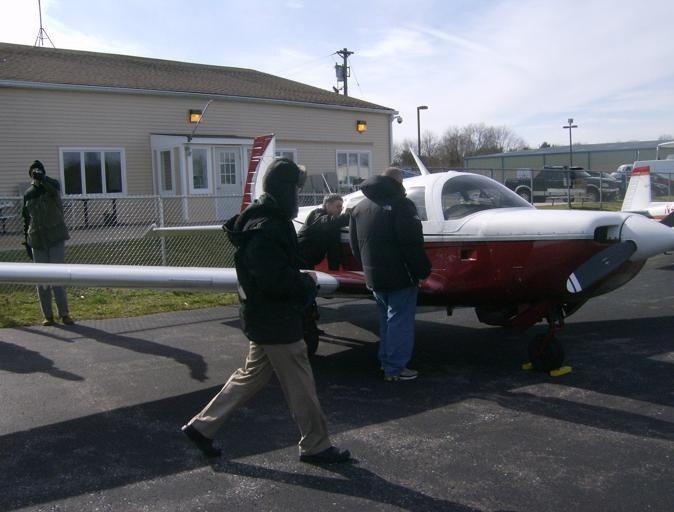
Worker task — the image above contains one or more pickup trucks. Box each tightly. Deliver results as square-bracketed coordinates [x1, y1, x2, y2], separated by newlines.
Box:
[505, 143, 673, 200]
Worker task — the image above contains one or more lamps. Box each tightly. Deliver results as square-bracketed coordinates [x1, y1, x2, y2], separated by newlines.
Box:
[189, 109, 203, 123]
[357, 120, 367, 133]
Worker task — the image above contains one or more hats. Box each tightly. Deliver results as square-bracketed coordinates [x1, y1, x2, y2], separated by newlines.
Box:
[29, 159, 45, 177]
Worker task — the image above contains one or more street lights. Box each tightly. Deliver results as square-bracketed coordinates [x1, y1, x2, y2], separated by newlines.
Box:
[417, 106, 428, 157]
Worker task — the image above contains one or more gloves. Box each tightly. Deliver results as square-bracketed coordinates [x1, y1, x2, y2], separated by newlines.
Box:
[32, 168, 46, 183]
[23, 242, 33, 259]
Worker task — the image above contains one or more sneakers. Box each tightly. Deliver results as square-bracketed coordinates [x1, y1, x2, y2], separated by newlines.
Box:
[42, 316, 73, 325]
[381, 362, 419, 382]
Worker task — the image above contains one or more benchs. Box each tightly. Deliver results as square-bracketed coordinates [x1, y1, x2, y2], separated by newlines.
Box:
[547, 188, 591, 206]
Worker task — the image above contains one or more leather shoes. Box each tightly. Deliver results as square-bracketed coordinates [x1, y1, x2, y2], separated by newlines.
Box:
[182, 422, 223, 456]
[300, 446, 351, 463]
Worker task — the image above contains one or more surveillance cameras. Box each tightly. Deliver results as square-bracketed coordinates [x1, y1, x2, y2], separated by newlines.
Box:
[397, 117, 403, 124]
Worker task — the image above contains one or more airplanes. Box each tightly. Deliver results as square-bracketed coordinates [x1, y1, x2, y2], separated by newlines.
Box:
[0, 133, 673, 377]
[619, 164, 674, 228]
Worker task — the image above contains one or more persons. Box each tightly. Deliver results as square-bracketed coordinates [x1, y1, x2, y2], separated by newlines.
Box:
[22, 159, 74, 325]
[348, 167, 433, 384]
[178, 156, 352, 463]
[294, 192, 350, 270]
